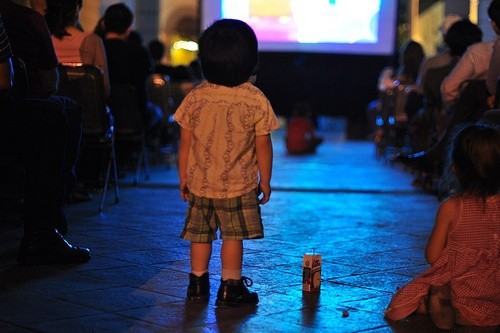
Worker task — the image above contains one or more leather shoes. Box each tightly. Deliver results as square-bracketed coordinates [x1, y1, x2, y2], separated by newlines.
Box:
[186, 271, 210, 302]
[215, 275, 259, 306]
[14, 230, 94, 263]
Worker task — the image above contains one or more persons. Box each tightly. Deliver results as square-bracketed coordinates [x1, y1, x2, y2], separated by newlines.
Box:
[172, 18, 280, 307]
[0, 0, 201, 271]
[382, 126, 500, 333]
[369, 0, 500, 193]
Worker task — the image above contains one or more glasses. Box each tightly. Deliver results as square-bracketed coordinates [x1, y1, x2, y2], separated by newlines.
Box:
[488, 17, 493, 23]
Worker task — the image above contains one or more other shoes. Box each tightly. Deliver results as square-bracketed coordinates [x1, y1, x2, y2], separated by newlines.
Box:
[428, 283, 456, 330]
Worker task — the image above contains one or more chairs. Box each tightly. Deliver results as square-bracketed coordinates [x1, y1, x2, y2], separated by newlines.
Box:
[106, 78, 160, 186]
[449, 79, 490, 122]
[7, 51, 41, 100]
[60, 60, 123, 212]
[493, 76, 500, 114]
